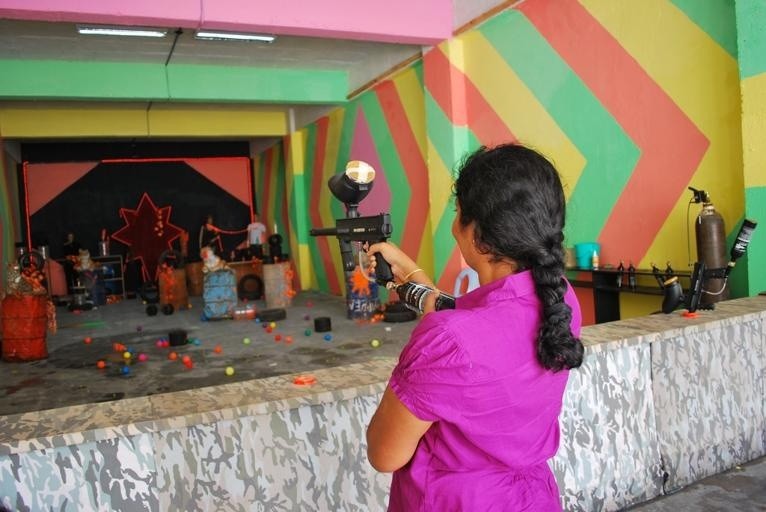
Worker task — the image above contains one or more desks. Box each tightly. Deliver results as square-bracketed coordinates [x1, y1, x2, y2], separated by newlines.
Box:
[564, 265, 693, 324]
[230, 259, 263, 292]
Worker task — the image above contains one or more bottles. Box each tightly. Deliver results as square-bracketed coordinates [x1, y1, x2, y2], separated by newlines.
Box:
[650, 263, 666, 290]
[39, 243, 49, 261]
[628, 260, 636, 289]
[14, 242, 27, 258]
[387, 280, 458, 317]
[615, 259, 624, 288]
[592, 251, 600, 268]
[666, 260, 673, 281]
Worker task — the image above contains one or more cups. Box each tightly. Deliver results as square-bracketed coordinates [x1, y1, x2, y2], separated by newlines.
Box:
[96, 241, 110, 256]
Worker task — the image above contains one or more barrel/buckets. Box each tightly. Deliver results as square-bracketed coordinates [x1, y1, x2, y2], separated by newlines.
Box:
[262, 255, 293, 309]
[2, 288, 50, 362]
[158, 265, 189, 311]
[572, 242, 600, 270]
[202, 267, 238, 322]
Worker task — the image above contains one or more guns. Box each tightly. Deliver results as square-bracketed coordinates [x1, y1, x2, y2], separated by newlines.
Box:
[310, 212, 393, 282]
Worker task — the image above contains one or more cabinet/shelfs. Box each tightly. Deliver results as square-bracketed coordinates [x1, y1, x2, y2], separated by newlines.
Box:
[92, 255, 126, 300]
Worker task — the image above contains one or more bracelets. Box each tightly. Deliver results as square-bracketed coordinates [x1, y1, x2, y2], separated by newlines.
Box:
[403, 269, 423, 280]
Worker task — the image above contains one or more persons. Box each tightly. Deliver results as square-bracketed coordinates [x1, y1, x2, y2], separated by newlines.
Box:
[362, 140, 584, 512]
[61, 232, 78, 259]
[247, 214, 266, 259]
